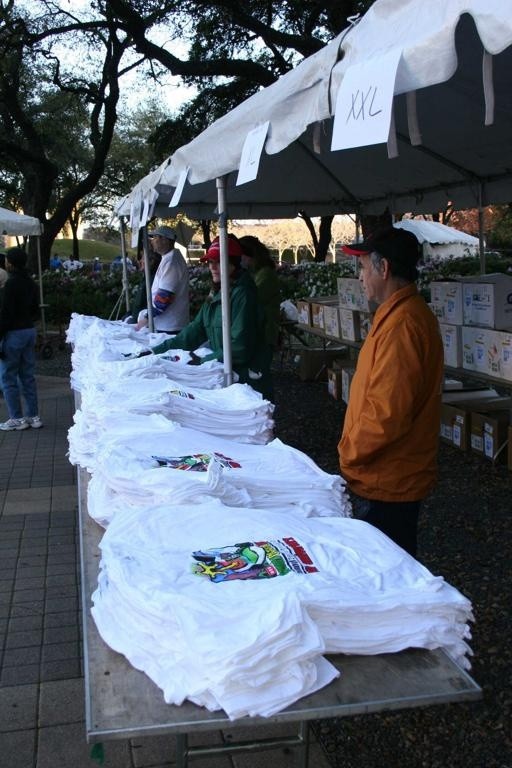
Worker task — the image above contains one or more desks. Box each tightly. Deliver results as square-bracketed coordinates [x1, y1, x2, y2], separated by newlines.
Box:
[69, 343, 482, 767]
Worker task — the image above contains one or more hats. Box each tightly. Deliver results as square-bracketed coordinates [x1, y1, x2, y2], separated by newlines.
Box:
[341, 227, 418, 255]
[199, 234, 242, 262]
[148, 226, 177, 240]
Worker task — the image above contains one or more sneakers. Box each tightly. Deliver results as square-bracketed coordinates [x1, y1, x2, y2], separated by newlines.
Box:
[0, 417, 29, 431]
[24, 416, 42, 428]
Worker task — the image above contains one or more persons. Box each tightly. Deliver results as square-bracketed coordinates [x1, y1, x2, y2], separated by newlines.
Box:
[0, 247, 44, 432]
[49, 251, 145, 276]
[134, 224, 194, 338]
[1, 230, 14, 290]
[334, 225, 444, 558]
[122, 248, 160, 326]
[122, 232, 273, 401]
[238, 234, 279, 349]
